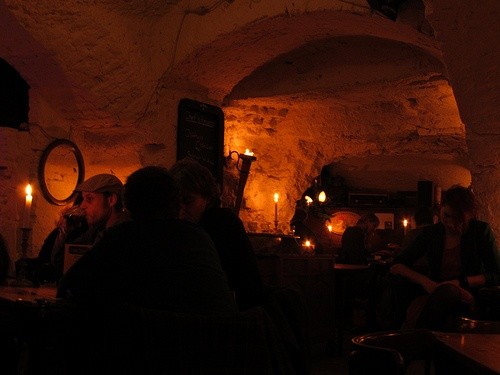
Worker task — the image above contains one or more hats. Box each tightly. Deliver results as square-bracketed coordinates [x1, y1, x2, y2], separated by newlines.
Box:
[76, 173, 123, 193]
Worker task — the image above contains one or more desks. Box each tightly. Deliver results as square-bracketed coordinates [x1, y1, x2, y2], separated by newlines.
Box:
[430, 329, 500, 375]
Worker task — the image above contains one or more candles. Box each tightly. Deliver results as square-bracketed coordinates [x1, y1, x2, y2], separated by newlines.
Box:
[275, 202, 278, 221]
[24, 195, 33, 228]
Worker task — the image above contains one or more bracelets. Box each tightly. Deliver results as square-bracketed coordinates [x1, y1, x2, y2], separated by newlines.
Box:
[459, 276, 469, 290]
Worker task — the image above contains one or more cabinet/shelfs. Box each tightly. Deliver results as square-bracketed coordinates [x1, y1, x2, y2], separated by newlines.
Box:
[260, 254, 336, 347]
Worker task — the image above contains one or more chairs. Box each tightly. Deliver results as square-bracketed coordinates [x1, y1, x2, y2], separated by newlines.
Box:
[347, 326, 433, 375]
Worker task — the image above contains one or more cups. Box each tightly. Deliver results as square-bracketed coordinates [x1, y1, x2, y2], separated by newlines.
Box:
[63, 205, 86, 228]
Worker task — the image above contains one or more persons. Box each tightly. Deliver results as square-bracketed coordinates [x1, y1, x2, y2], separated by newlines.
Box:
[168, 159, 315, 375]
[50, 174, 129, 274]
[55, 166, 220, 338]
[389, 187, 500, 334]
[342, 213, 381, 267]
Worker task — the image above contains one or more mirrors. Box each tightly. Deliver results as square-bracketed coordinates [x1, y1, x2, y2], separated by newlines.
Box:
[39, 139, 86, 205]
